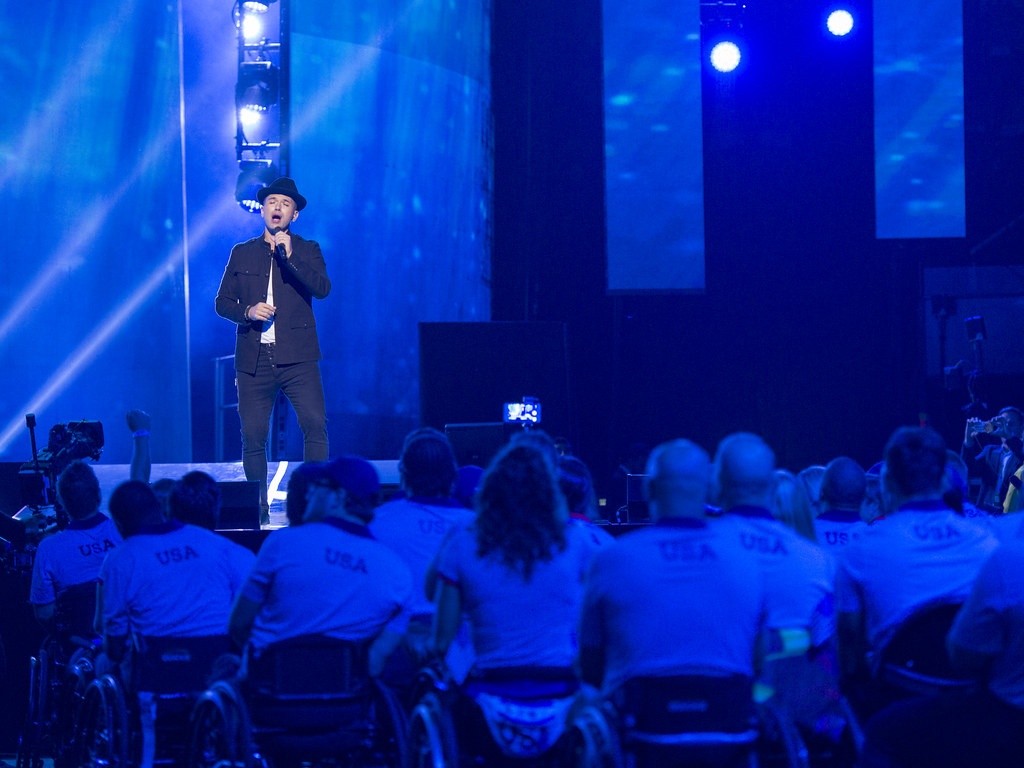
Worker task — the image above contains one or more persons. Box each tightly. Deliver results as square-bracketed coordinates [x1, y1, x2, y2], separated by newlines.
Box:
[214, 178, 330, 523]
[0, 407, 1024, 767]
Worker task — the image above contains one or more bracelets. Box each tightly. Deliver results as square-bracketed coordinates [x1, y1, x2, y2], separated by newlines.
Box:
[133, 429, 150, 439]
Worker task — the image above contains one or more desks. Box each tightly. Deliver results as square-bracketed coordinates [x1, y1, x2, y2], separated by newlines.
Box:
[209, 354, 275, 462]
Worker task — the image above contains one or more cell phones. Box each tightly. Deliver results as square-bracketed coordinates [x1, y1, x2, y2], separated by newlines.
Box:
[504, 401, 541, 426]
[974, 422, 992, 433]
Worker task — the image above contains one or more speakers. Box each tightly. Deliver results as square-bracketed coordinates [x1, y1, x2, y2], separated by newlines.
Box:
[0, 462, 55, 515]
[417, 319, 575, 459]
[217, 479, 262, 530]
[447, 422, 514, 468]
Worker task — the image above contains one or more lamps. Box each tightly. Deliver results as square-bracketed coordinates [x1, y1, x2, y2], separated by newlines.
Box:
[239, 81, 278, 115]
[240, 0, 277, 11]
[235, 166, 269, 214]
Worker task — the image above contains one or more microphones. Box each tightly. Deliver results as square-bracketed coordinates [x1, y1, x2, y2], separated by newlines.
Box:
[275, 228, 287, 259]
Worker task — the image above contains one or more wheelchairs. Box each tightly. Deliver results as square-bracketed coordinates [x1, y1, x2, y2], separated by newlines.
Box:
[12, 631, 804, 768]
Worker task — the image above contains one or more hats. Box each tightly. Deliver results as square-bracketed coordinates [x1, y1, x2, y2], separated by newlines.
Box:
[257, 176, 307, 212]
